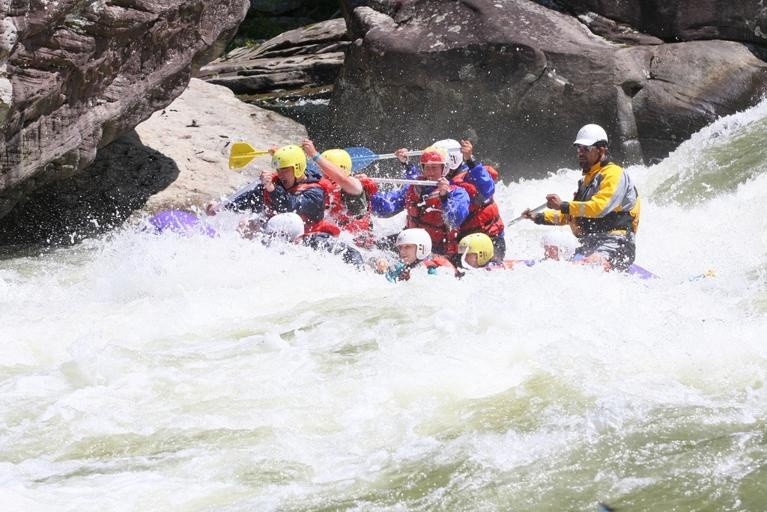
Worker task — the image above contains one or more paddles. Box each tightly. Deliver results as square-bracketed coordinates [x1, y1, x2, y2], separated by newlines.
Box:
[343, 147, 424, 173]
[228, 142, 303, 170]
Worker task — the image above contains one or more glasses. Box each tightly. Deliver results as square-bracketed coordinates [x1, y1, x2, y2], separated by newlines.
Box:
[576, 145, 596, 153]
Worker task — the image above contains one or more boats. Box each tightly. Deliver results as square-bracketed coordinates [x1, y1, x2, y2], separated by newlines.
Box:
[141, 211, 662, 283]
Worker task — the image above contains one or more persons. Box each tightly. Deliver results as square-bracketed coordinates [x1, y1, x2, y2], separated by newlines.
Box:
[205, 138, 506, 285]
[521, 122, 641, 270]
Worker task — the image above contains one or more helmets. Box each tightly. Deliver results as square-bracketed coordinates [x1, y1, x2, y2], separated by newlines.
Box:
[458, 232, 494, 266]
[395, 228, 432, 260]
[320, 149, 352, 176]
[268, 212, 304, 241]
[573, 123, 609, 146]
[270, 145, 306, 177]
[420, 139, 463, 177]
